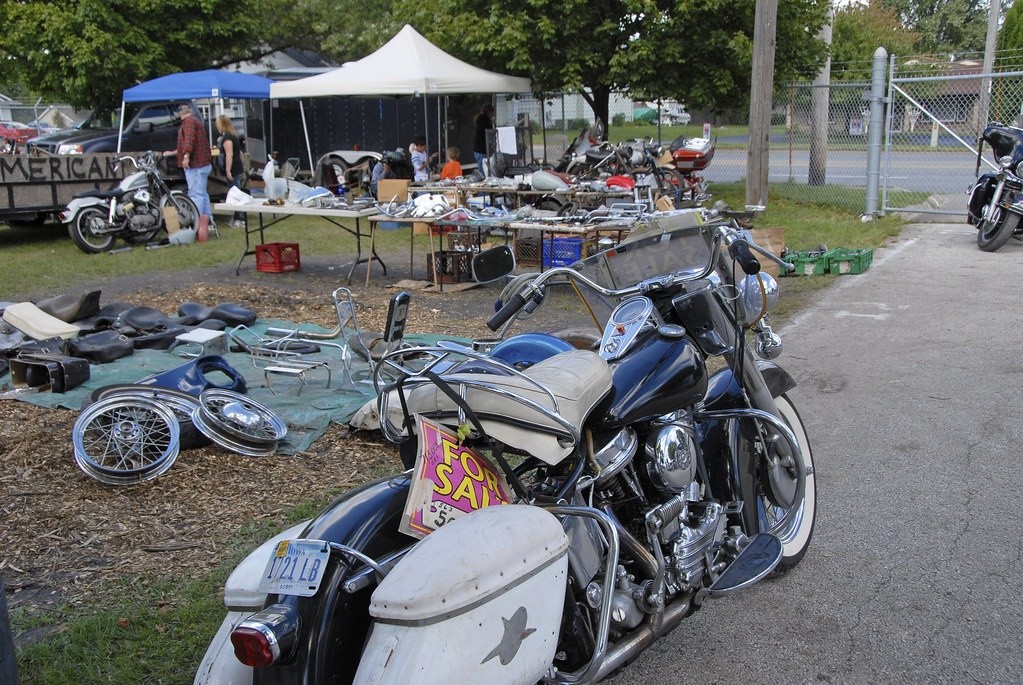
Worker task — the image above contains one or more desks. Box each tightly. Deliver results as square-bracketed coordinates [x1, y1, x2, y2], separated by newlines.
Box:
[575, 191, 635, 210]
[458, 186, 519, 209]
[214, 197, 387, 286]
[365, 214, 440, 292]
[516, 189, 575, 208]
[434, 210, 598, 257]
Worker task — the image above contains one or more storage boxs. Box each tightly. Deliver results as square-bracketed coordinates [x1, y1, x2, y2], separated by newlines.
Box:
[255, 242, 302, 273]
[379, 202, 412, 230]
[447, 229, 484, 250]
[543, 237, 582, 267]
[720, 227, 786, 282]
[790, 248, 836, 276]
[377, 178, 412, 202]
[426, 250, 473, 284]
[827, 248, 874, 276]
[467, 195, 491, 211]
[779, 250, 798, 278]
[428, 222, 457, 236]
[516, 237, 541, 267]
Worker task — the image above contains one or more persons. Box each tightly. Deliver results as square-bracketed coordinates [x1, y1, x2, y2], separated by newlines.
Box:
[371, 146, 414, 202]
[215, 115, 245, 228]
[408, 136, 439, 183]
[439, 146, 462, 180]
[177, 104, 214, 231]
[473, 103, 495, 197]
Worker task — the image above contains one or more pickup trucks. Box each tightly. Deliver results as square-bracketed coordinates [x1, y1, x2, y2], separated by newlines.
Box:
[26, 100, 251, 155]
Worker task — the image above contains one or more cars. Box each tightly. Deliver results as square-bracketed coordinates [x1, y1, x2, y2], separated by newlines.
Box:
[0, 120, 40, 145]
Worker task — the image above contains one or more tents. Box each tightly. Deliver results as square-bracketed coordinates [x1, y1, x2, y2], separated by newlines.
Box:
[117, 69, 315, 178]
[270, 24, 540, 181]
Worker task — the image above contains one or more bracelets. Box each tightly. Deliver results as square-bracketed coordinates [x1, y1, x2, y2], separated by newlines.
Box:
[184, 155, 189, 159]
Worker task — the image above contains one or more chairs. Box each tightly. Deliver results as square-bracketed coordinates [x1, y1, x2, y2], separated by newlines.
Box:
[333, 287, 387, 397]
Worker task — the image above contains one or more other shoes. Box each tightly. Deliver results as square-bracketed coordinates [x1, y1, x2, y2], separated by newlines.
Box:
[227, 217, 249, 228]
[208, 225, 214, 231]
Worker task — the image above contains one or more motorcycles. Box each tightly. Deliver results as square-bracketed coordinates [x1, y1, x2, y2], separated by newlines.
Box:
[966, 115, 1023, 251]
[543, 115, 718, 218]
[193, 208, 819, 685]
[58, 149, 201, 253]
